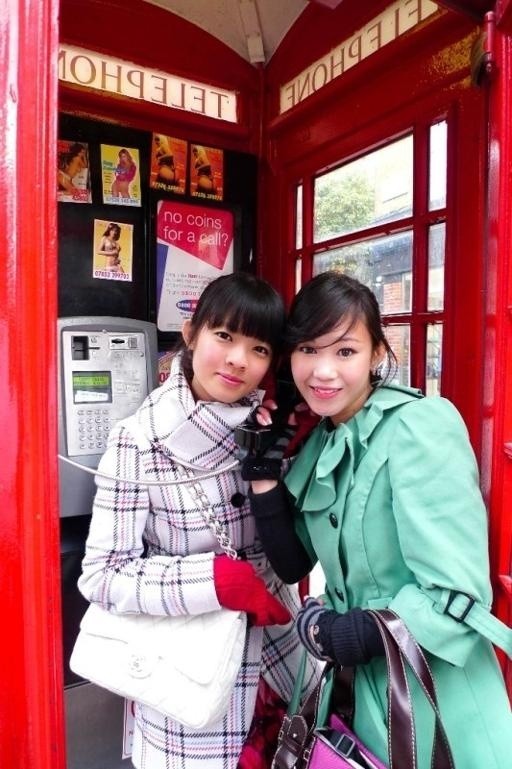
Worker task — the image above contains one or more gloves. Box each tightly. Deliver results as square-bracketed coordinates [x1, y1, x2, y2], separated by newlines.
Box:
[215, 555, 292, 626]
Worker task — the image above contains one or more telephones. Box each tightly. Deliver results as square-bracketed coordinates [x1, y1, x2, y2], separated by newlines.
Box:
[234, 358, 300, 451]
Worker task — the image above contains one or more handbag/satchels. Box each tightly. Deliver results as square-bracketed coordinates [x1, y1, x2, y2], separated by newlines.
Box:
[68, 603, 249, 728]
[272, 705, 388, 768]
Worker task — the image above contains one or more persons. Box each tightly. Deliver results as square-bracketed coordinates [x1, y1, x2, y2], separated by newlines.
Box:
[111, 149, 136, 198]
[77, 272, 326, 768]
[98, 223, 124, 272]
[192, 145, 216, 195]
[57, 143, 88, 193]
[154, 133, 179, 191]
[241, 270, 512, 769]
